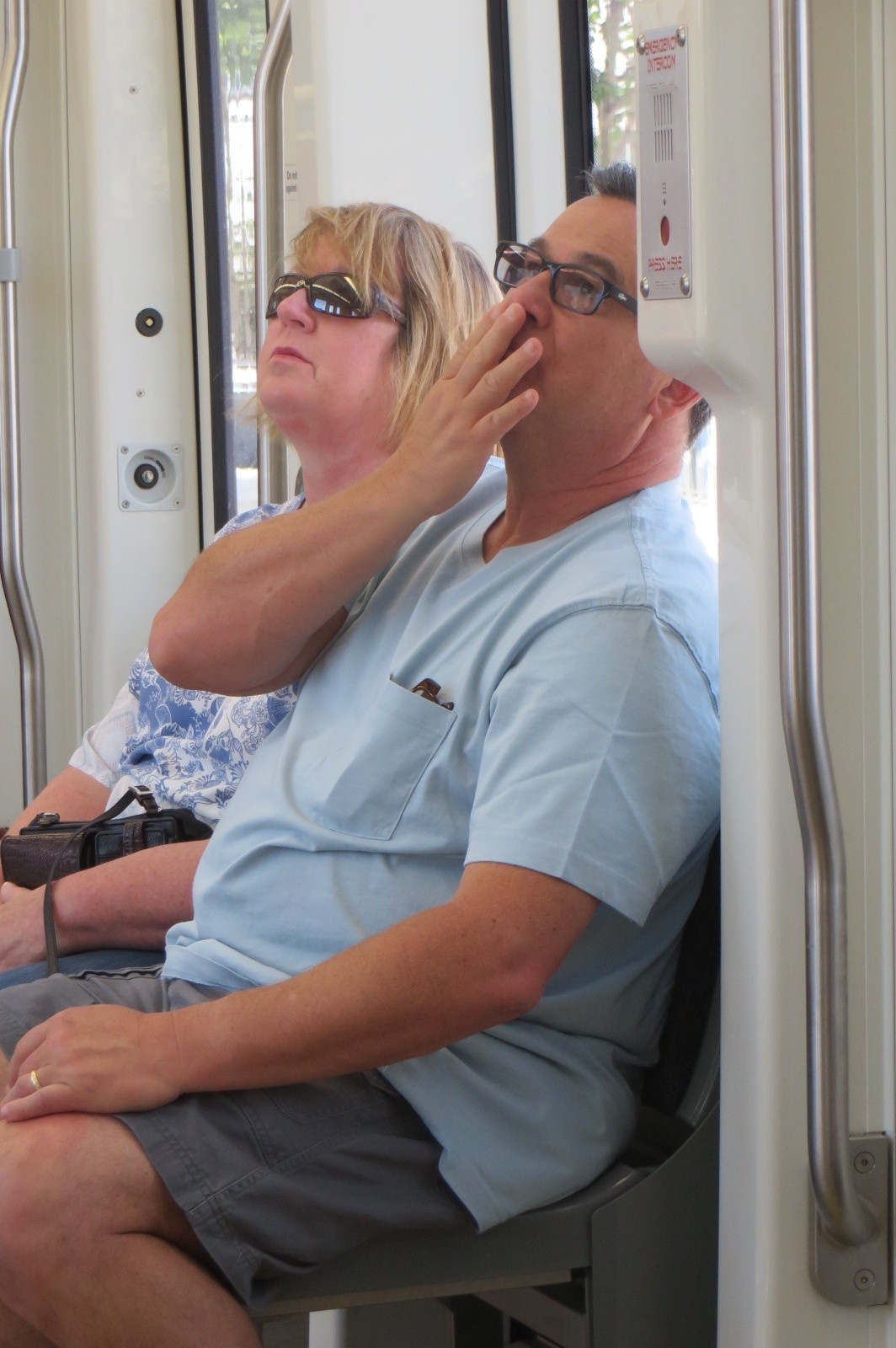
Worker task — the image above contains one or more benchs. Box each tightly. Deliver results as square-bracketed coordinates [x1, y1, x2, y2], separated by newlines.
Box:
[231, 836, 725, 1347]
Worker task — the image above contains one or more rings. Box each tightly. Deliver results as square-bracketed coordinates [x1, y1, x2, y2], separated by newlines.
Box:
[29, 1068, 43, 1091]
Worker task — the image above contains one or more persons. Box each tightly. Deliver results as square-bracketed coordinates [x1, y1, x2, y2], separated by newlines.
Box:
[1, 169, 723, 1347]
[0, 202, 506, 983]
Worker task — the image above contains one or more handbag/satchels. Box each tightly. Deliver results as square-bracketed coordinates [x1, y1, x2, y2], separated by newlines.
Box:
[2, 786, 217, 892]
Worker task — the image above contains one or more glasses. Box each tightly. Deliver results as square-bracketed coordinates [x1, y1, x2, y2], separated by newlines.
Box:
[265, 271, 407, 328]
[493, 239, 637, 316]
[411, 678, 454, 711]
